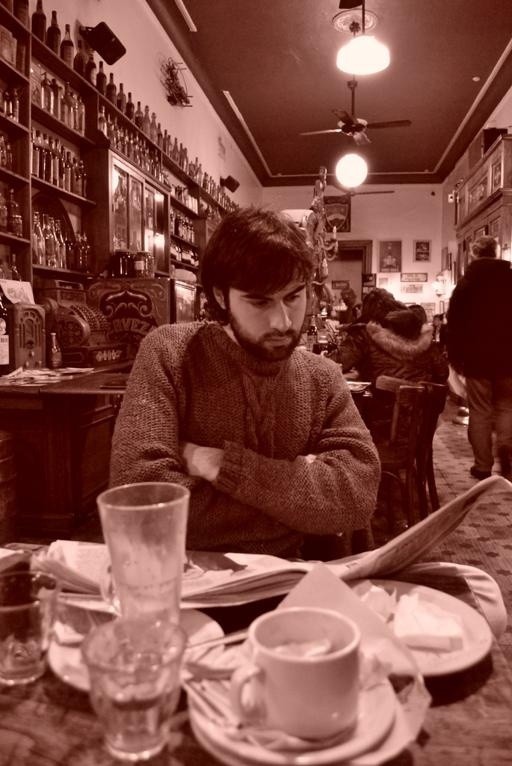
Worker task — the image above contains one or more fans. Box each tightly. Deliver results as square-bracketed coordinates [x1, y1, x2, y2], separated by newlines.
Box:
[299, 80, 411, 147]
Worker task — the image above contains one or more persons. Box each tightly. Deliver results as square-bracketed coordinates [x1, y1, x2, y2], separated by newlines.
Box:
[109, 206, 382, 559]
[323, 287, 449, 443]
[446, 236, 512, 481]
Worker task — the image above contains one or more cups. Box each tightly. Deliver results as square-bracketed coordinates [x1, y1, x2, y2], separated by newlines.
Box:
[93, 482, 192, 620]
[81, 622, 188, 762]
[232, 606, 360, 739]
[0, 568, 57, 688]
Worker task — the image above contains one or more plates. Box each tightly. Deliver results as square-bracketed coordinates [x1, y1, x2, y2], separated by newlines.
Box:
[199, 647, 396, 760]
[351, 577, 494, 679]
[46, 606, 223, 695]
[346, 380, 370, 392]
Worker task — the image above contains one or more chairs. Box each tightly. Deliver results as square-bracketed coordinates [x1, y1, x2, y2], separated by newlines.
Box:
[369, 375, 448, 546]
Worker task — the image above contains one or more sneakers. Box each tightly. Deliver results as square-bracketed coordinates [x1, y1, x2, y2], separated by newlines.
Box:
[471, 468, 491, 480]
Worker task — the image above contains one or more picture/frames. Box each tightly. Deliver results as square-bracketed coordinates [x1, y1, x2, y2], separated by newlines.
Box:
[441, 246, 452, 271]
[377, 239, 403, 275]
[414, 238, 432, 263]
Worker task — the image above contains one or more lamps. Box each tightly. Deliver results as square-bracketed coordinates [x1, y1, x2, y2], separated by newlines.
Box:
[220, 175, 240, 192]
[336, 0, 391, 76]
[335, 140, 369, 189]
[79, 20, 126, 65]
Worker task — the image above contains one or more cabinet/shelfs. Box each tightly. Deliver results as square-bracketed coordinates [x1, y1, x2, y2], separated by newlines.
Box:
[0, 1, 231, 371]
[454, 133, 512, 284]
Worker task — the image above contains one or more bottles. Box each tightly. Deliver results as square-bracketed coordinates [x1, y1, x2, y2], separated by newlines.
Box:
[1, 0, 236, 282]
[305, 318, 317, 350]
[1, 302, 12, 375]
[48, 333, 63, 369]
